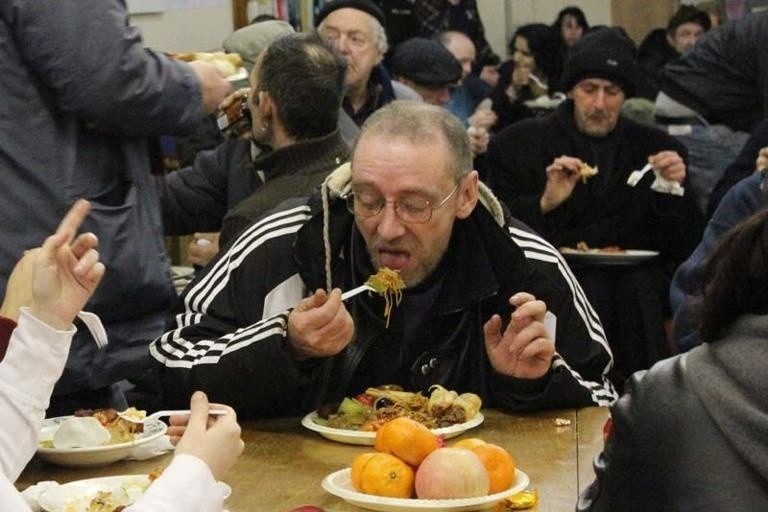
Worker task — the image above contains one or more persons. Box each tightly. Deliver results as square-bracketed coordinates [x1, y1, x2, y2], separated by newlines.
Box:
[1, 1, 767, 414]
[575, 209, 767, 510]
[0, 199, 244, 512]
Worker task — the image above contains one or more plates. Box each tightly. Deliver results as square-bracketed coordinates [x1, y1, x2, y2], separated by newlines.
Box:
[558, 245, 659, 268]
[298, 405, 485, 448]
[33, 414, 168, 469]
[35, 473, 233, 512]
[526, 97, 561, 110]
[318, 465, 532, 511]
[167, 265, 195, 278]
[170, 53, 249, 83]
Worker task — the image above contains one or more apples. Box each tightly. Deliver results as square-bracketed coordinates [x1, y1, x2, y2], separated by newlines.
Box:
[414, 446, 490, 498]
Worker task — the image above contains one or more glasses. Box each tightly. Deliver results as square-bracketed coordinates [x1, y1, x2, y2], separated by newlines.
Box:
[340, 188, 457, 224]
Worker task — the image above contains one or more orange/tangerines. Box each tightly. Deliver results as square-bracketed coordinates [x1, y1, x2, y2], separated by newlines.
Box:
[351, 418, 436, 499]
[456, 438, 514, 494]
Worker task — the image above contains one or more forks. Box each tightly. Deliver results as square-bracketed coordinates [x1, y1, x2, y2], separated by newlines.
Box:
[76, 308, 114, 350]
[528, 73, 547, 89]
[338, 272, 394, 305]
[115, 408, 229, 425]
[624, 160, 653, 186]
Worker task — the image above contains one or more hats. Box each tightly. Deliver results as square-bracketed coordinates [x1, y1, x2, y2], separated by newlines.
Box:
[562, 27, 638, 95]
[395, 37, 462, 86]
